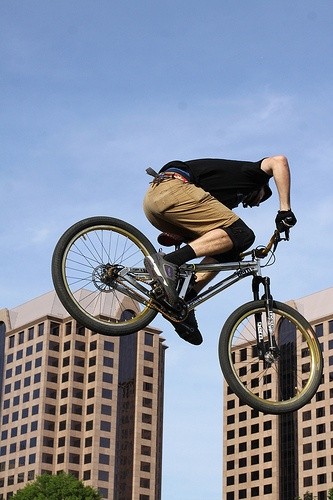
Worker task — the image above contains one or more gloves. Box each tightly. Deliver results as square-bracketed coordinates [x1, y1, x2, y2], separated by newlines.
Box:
[275, 209, 297, 233]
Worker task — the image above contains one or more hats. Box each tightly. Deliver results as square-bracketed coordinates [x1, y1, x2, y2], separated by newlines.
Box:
[261, 180, 272, 203]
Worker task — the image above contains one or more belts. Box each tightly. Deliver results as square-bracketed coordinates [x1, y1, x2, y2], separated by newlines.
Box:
[153, 174, 188, 183]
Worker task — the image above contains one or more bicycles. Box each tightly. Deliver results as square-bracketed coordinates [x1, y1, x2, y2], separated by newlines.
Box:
[51, 216, 324, 415]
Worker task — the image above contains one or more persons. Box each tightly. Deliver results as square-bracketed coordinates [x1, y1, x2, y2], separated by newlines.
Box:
[143, 155, 297, 345]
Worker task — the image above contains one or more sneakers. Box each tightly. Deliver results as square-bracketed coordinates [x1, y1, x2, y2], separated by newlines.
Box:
[144, 248, 183, 308]
[162, 307, 203, 345]
